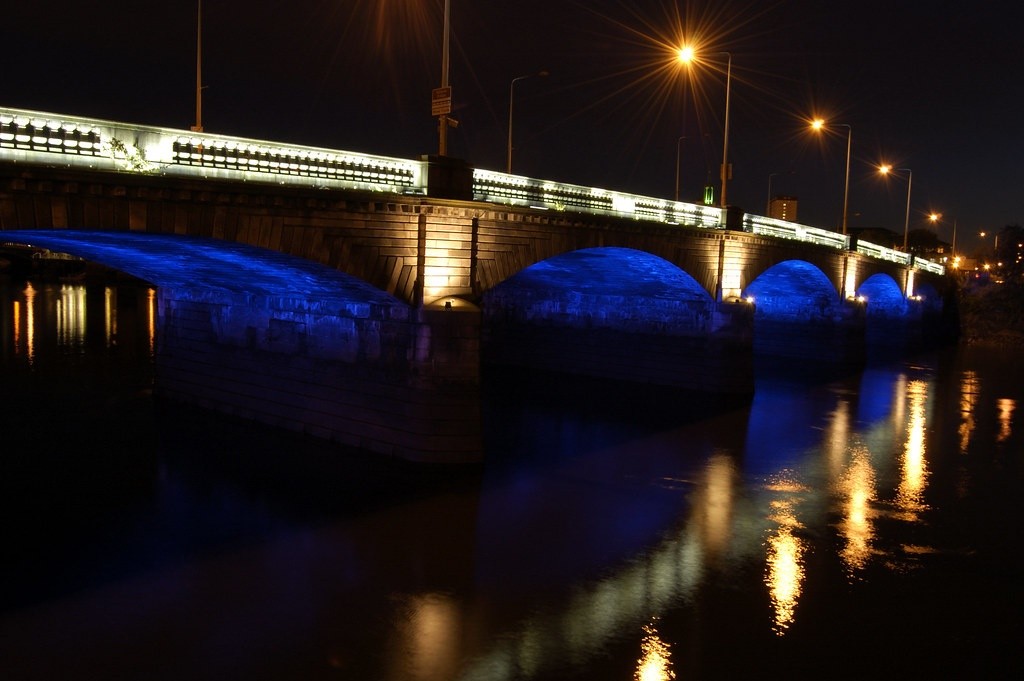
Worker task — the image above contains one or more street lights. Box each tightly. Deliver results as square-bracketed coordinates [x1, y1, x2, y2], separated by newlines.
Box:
[931, 212, 957, 259]
[681, 44, 731, 207]
[507, 71, 549, 174]
[810, 118, 852, 236]
[879, 165, 913, 253]
